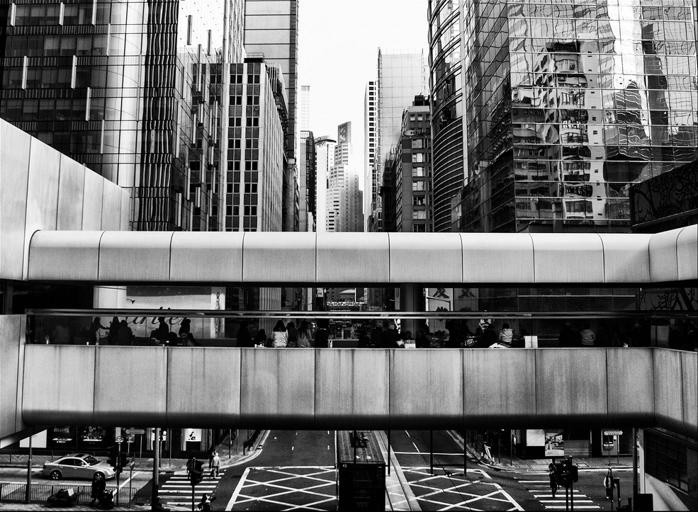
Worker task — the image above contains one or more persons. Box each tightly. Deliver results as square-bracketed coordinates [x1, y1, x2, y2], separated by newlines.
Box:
[206, 448, 216, 470]
[211, 452, 219, 479]
[603, 471, 617, 501]
[199, 493, 215, 512]
[547, 458, 559, 500]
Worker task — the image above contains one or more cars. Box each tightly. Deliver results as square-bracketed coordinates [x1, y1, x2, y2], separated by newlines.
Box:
[43, 452, 116, 481]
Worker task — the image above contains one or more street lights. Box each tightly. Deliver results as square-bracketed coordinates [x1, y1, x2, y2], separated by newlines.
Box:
[115, 436, 124, 505]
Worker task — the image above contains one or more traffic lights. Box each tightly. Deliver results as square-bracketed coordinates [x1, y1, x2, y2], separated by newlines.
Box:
[194, 462, 204, 485]
